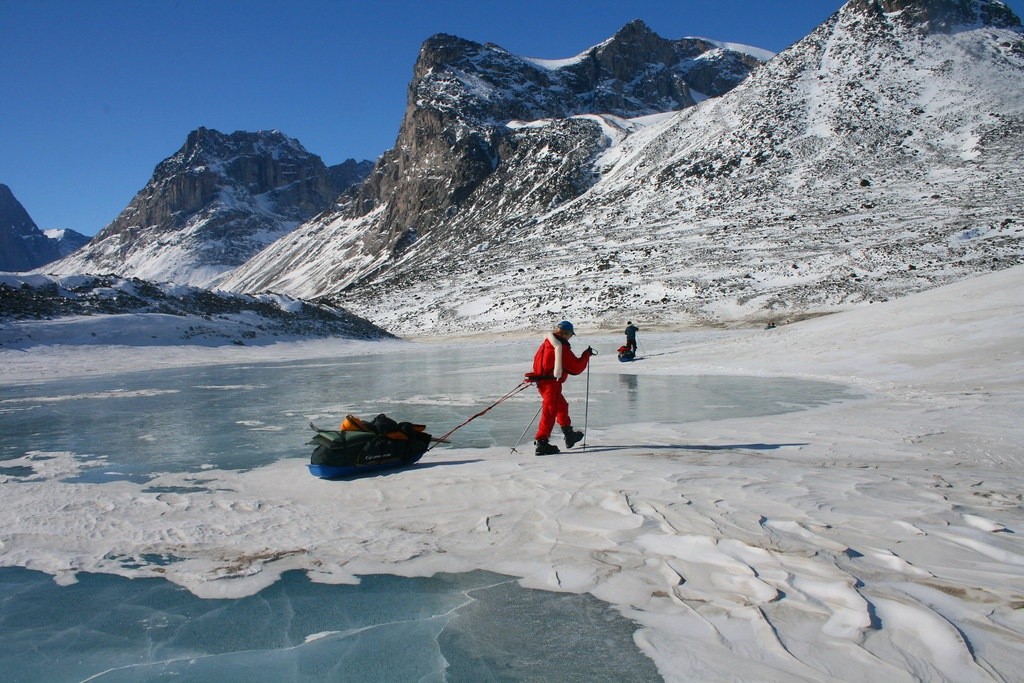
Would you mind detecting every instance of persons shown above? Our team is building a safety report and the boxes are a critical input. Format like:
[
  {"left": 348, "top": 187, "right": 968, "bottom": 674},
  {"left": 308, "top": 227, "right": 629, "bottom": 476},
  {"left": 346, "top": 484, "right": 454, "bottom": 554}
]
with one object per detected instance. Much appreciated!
[
  {"left": 767, "top": 323, "right": 776, "bottom": 329},
  {"left": 533, "top": 322, "right": 593, "bottom": 455},
  {"left": 625, "top": 321, "right": 639, "bottom": 357}
]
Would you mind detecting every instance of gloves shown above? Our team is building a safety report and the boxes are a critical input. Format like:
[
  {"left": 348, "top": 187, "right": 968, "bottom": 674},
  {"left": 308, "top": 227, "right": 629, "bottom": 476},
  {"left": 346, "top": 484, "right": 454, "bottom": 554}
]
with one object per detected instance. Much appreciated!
[{"left": 588, "top": 345, "right": 593, "bottom": 356}]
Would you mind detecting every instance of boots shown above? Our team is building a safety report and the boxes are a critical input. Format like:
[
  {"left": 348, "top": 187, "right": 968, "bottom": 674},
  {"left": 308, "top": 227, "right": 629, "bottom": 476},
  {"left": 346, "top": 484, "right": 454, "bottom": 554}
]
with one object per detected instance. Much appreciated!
[
  {"left": 534, "top": 439, "right": 560, "bottom": 455},
  {"left": 561, "top": 424, "right": 584, "bottom": 448}
]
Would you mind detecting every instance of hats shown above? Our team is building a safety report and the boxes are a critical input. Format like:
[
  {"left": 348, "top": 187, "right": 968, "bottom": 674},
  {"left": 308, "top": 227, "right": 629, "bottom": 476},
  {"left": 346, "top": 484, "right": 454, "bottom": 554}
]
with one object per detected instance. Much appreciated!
[{"left": 557, "top": 321, "right": 576, "bottom": 336}]
